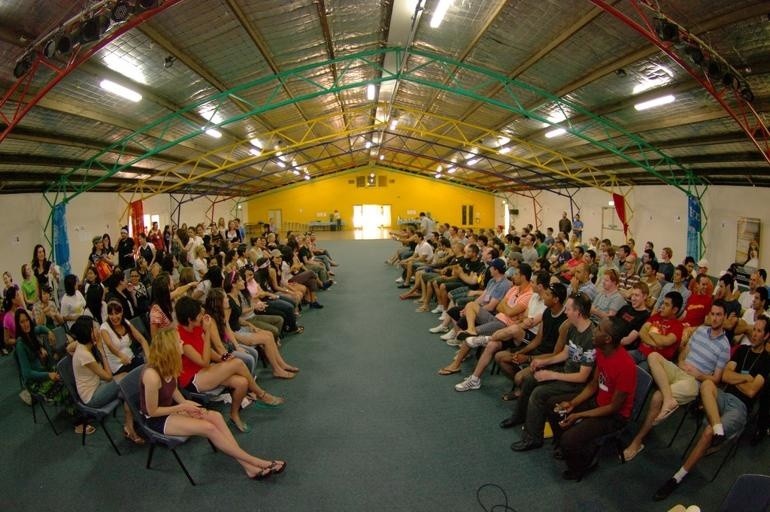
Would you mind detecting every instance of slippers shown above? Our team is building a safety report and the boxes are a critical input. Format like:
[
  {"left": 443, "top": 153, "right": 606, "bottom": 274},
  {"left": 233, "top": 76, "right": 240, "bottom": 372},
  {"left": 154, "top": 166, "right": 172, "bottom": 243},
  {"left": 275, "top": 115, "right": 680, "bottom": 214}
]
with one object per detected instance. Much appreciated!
[
  {"left": 457, "top": 331, "right": 478, "bottom": 341},
  {"left": 619, "top": 444, "right": 645, "bottom": 462},
  {"left": 285, "top": 366, "right": 299, "bottom": 372},
  {"left": 655, "top": 401, "right": 680, "bottom": 422},
  {"left": 439, "top": 367, "right": 462, "bottom": 376},
  {"left": 272, "top": 372, "right": 297, "bottom": 379},
  {"left": 254, "top": 468, "right": 273, "bottom": 481},
  {"left": 265, "top": 460, "right": 287, "bottom": 473}
]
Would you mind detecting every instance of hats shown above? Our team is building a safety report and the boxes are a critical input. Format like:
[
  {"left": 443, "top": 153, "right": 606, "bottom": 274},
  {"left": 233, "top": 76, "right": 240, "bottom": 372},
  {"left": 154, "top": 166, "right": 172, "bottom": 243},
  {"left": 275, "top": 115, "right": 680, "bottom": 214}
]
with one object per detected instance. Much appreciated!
[
  {"left": 487, "top": 259, "right": 507, "bottom": 272},
  {"left": 697, "top": 258, "right": 709, "bottom": 269},
  {"left": 505, "top": 252, "right": 524, "bottom": 263},
  {"left": 271, "top": 249, "right": 284, "bottom": 260}
]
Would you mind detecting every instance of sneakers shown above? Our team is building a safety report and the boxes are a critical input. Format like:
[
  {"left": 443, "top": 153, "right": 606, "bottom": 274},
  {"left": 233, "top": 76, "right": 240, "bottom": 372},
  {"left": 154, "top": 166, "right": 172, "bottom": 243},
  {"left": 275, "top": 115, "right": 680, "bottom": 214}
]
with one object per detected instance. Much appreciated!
[
  {"left": 466, "top": 336, "right": 490, "bottom": 349},
  {"left": 448, "top": 339, "right": 465, "bottom": 347},
  {"left": 19, "top": 390, "right": 33, "bottom": 406},
  {"left": 416, "top": 304, "right": 448, "bottom": 321},
  {"left": 455, "top": 375, "right": 481, "bottom": 392},
  {"left": 395, "top": 278, "right": 404, "bottom": 283},
  {"left": 429, "top": 325, "right": 450, "bottom": 334},
  {"left": 440, "top": 330, "right": 458, "bottom": 341}
]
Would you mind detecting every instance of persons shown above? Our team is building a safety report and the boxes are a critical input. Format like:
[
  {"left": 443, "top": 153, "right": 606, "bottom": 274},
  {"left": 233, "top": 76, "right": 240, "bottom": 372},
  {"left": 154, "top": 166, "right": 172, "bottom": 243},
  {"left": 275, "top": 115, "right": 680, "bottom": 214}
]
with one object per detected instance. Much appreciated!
[
  {"left": 1, "top": 217, "right": 338, "bottom": 479},
  {"left": 385, "top": 208, "right": 769, "bottom": 500}
]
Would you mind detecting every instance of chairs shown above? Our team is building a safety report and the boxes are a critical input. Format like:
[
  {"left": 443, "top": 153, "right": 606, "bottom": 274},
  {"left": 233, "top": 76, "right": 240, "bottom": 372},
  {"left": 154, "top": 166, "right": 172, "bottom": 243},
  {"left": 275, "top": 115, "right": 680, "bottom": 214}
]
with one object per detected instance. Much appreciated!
[
  {"left": 56, "top": 355, "right": 126, "bottom": 457},
  {"left": 715, "top": 471, "right": 770, "bottom": 512},
  {"left": 119, "top": 364, "right": 215, "bottom": 487},
  {"left": 12, "top": 345, "right": 76, "bottom": 436}
]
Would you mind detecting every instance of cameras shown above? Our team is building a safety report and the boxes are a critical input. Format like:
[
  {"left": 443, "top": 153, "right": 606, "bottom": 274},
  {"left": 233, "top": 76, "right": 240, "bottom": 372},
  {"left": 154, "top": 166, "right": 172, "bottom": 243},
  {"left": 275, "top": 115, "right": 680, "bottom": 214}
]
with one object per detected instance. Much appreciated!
[{"left": 559, "top": 410, "right": 567, "bottom": 421}]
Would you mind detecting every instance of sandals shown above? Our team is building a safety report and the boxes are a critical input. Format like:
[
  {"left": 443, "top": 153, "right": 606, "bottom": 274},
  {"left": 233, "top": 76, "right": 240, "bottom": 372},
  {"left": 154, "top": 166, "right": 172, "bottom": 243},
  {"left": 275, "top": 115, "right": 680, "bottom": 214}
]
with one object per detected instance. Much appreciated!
[
  {"left": 257, "top": 392, "right": 286, "bottom": 407},
  {"left": 123, "top": 426, "right": 146, "bottom": 445},
  {"left": 76, "top": 425, "right": 97, "bottom": 435},
  {"left": 230, "top": 418, "right": 252, "bottom": 434}
]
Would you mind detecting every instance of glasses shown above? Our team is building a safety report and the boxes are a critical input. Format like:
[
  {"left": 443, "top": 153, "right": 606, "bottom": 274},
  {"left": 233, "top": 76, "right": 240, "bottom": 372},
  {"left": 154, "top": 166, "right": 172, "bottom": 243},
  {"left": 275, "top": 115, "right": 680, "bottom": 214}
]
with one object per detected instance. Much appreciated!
[{"left": 611, "top": 269, "right": 617, "bottom": 277}]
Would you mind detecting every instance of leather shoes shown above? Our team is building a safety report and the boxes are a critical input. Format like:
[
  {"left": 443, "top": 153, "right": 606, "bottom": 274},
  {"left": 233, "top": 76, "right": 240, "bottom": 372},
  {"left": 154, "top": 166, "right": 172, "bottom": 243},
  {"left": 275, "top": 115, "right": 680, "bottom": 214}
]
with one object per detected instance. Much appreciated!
[
  {"left": 654, "top": 478, "right": 681, "bottom": 501},
  {"left": 555, "top": 452, "right": 566, "bottom": 461},
  {"left": 398, "top": 284, "right": 410, "bottom": 288},
  {"left": 511, "top": 439, "right": 544, "bottom": 452},
  {"left": 562, "top": 470, "right": 581, "bottom": 481},
  {"left": 310, "top": 302, "right": 323, "bottom": 309},
  {"left": 712, "top": 431, "right": 728, "bottom": 448},
  {"left": 500, "top": 418, "right": 516, "bottom": 429},
  {"left": 320, "top": 280, "right": 333, "bottom": 290}
]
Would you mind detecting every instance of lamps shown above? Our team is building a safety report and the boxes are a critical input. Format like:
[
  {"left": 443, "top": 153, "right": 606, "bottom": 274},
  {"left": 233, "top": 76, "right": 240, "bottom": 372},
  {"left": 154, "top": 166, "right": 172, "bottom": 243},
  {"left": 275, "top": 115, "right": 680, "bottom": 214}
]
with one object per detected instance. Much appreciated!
[
  {"left": 164, "top": 55, "right": 177, "bottom": 68},
  {"left": 12, "top": 0, "right": 167, "bottom": 78},
  {"left": 652, "top": 12, "right": 754, "bottom": 102}
]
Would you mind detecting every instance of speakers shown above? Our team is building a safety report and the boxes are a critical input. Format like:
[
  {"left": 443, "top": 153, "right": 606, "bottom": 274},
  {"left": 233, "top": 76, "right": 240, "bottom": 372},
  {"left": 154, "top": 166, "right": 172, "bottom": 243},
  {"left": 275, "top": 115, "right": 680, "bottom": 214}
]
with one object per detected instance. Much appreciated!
[{"left": 509, "top": 209, "right": 519, "bottom": 215}]
